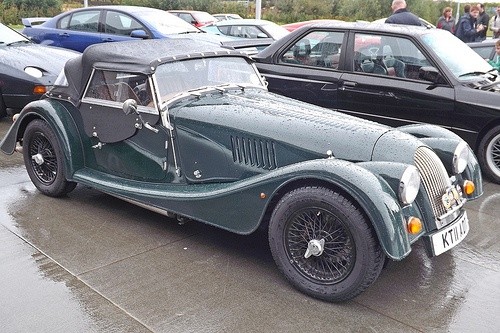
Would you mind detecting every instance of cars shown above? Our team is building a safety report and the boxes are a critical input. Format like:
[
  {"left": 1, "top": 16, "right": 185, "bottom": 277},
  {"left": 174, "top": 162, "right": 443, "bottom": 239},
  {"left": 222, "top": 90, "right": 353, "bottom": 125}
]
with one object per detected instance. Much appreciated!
[
  {"left": 22, "top": 5, "right": 259, "bottom": 54},
  {"left": 200, "top": 18, "right": 318, "bottom": 51},
  {"left": 252, "top": 21, "right": 500, "bottom": 186},
  {"left": 213, "top": 14, "right": 244, "bottom": 22},
  {"left": 0, "top": 22, "right": 83, "bottom": 119},
  {"left": 164, "top": 9, "right": 218, "bottom": 27},
  {"left": 0, "top": 38, "right": 485, "bottom": 304},
  {"left": 281, "top": 19, "right": 500, "bottom": 64}
]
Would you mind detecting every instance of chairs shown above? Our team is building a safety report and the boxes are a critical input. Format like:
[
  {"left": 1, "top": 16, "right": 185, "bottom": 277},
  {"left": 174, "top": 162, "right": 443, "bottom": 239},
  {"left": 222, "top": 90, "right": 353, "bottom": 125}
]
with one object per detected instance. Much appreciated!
[
  {"left": 95, "top": 82, "right": 142, "bottom": 106},
  {"left": 359, "top": 45, "right": 406, "bottom": 79}
]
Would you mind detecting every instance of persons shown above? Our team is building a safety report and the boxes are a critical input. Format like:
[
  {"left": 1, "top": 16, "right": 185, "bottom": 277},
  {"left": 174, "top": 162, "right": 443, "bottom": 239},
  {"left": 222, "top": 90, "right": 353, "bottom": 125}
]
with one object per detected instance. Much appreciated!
[
  {"left": 380, "top": 0, "right": 421, "bottom": 61},
  {"left": 454, "top": 3, "right": 489, "bottom": 44},
  {"left": 489, "top": 6, "right": 500, "bottom": 39},
  {"left": 485, "top": 40, "right": 500, "bottom": 72},
  {"left": 436, "top": 7, "right": 455, "bottom": 35}
]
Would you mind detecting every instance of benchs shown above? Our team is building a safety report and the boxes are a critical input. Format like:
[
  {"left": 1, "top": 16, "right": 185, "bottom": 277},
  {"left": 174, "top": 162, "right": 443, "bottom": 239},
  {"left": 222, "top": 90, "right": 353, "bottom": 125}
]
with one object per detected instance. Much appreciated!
[{"left": 284, "top": 38, "right": 340, "bottom": 70}]
